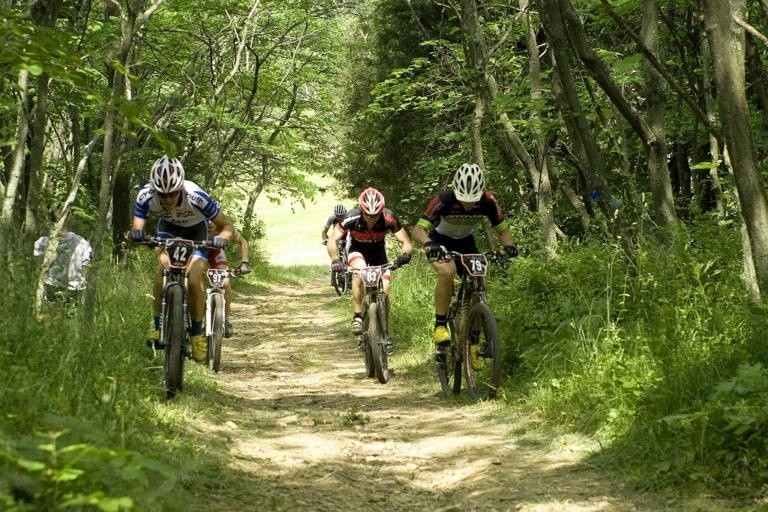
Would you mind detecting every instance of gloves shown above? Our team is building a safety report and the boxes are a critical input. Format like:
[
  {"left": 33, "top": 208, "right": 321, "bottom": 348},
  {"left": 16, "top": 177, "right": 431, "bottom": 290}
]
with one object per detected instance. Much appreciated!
[
  {"left": 235, "top": 262, "right": 252, "bottom": 276},
  {"left": 503, "top": 245, "right": 518, "bottom": 258},
  {"left": 397, "top": 253, "right": 411, "bottom": 265},
  {"left": 128, "top": 228, "right": 144, "bottom": 242},
  {"left": 212, "top": 236, "right": 224, "bottom": 248},
  {"left": 424, "top": 241, "right": 447, "bottom": 263},
  {"left": 332, "top": 259, "right": 344, "bottom": 272}
]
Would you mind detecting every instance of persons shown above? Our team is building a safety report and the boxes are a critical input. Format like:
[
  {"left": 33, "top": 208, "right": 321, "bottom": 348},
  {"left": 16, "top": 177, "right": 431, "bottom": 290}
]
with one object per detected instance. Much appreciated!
[
  {"left": 408, "top": 160, "right": 518, "bottom": 372},
  {"left": 320, "top": 202, "right": 351, "bottom": 281},
  {"left": 31, "top": 201, "right": 93, "bottom": 317},
  {"left": 327, "top": 186, "right": 413, "bottom": 356},
  {"left": 195, "top": 209, "right": 250, "bottom": 340},
  {"left": 125, "top": 155, "right": 235, "bottom": 364}
]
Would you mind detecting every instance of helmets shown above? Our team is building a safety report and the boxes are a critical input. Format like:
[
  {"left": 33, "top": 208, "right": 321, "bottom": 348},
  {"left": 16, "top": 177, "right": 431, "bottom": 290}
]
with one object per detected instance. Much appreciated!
[
  {"left": 451, "top": 163, "right": 486, "bottom": 203},
  {"left": 358, "top": 187, "right": 385, "bottom": 217},
  {"left": 150, "top": 154, "right": 185, "bottom": 194},
  {"left": 334, "top": 204, "right": 347, "bottom": 216}
]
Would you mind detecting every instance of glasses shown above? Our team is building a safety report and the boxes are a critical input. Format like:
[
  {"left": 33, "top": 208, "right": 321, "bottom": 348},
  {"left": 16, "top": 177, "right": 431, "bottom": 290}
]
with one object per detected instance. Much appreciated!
[{"left": 157, "top": 190, "right": 180, "bottom": 199}]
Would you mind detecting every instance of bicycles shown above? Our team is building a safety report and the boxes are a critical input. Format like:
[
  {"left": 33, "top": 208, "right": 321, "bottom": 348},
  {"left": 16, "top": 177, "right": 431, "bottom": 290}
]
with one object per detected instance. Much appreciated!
[
  {"left": 321, "top": 239, "right": 353, "bottom": 297},
  {"left": 333, "top": 257, "right": 402, "bottom": 383},
  {"left": 117, "top": 230, "right": 227, "bottom": 400},
  {"left": 417, "top": 243, "right": 515, "bottom": 397},
  {"left": 199, "top": 266, "right": 250, "bottom": 376}
]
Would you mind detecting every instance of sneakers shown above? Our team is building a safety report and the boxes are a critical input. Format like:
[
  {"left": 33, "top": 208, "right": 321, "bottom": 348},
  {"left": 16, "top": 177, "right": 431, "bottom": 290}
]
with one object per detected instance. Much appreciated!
[
  {"left": 191, "top": 334, "right": 208, "bottom": 362},
  {"left": 225, "top": 320, "right": 233, "bottom": 338},
  {"left": 434, "top": 326, "right": 451, "bottom": 346},
  {"left": 471, "top": 345, "right": 484, "bottom": 371},
  {"left": 147, "top": 330, "right": 166, "bottom": 350},
  {"left": 350, "top": 312, "right": 363, "bottom": 332}
]
